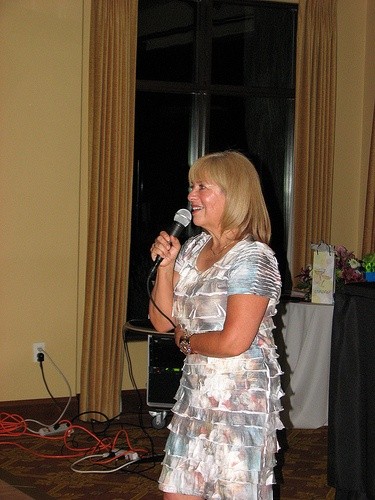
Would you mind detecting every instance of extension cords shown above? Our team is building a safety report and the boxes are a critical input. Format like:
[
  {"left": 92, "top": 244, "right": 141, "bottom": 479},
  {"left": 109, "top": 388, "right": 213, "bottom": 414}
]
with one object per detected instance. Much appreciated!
[
  {"left": 40, "top": 423, "right": 71, "bottom": 437},
  {"left": 111, "top": 448, "right": 138, "bottom": 461}
]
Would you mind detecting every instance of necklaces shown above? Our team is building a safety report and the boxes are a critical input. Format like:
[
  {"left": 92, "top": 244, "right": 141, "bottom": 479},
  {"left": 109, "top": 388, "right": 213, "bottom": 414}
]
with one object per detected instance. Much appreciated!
[{"left": 210, "top": 241, "right": 235, "bottom": 255}]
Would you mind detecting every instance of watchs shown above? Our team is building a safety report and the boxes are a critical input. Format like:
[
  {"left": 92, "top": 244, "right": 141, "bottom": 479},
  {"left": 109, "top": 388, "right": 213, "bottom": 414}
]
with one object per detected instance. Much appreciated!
[{"left": 179, "top": 331, "right": 194, "bottom": 355}]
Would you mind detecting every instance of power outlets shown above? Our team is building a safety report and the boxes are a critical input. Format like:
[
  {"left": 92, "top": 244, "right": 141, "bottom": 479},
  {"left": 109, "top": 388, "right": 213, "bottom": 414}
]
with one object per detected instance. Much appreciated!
[{"left": 33, "top": 343, "right": 47, "bottom": 363}]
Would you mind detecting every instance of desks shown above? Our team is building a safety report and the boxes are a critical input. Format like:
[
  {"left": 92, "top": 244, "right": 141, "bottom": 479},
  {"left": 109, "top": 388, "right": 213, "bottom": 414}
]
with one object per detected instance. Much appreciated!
[{"left": 273, "top": 299, "right": 329, "bottom": 429}]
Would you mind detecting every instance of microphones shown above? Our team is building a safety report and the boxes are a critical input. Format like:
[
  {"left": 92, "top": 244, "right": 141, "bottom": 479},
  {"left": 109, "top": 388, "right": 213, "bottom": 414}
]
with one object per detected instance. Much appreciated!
[{"left": 151, "top": 209, "right": 192, "bottom": 273}]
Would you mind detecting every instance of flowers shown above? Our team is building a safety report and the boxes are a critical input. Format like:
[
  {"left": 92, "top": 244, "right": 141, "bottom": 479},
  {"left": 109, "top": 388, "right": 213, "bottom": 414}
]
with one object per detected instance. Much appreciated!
[{"left": 294, "top": 238, "right": 375, "bottom": 302}]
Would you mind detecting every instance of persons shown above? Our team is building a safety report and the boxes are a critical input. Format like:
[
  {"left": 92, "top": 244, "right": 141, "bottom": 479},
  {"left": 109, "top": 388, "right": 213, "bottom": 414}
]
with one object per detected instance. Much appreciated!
[{"left": 151, "top": 153, "right": 286, "bottom": 500}]
